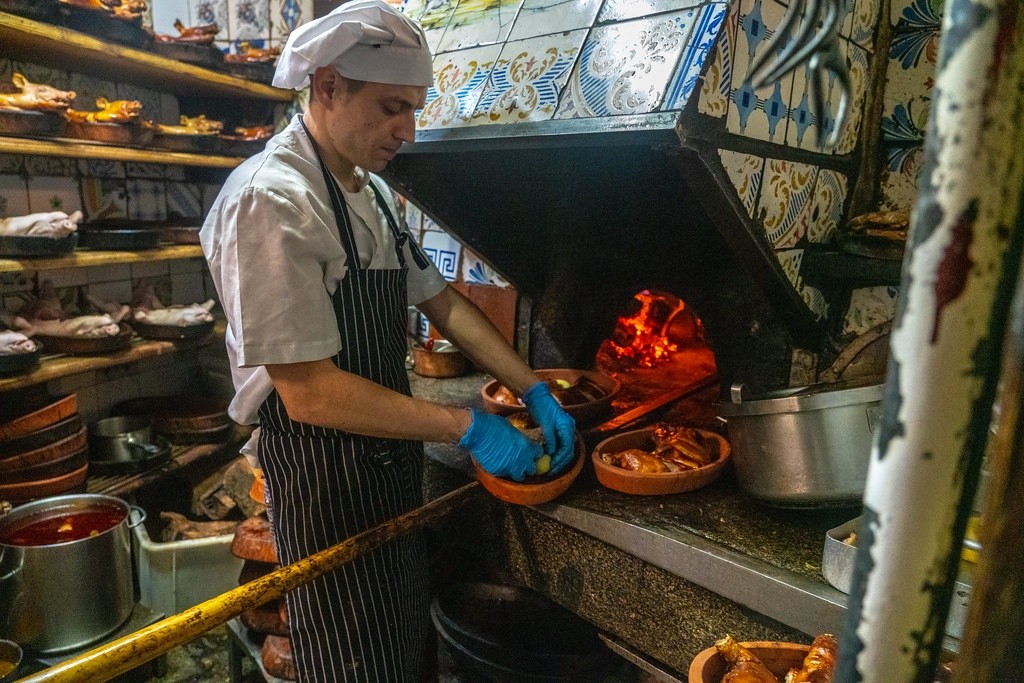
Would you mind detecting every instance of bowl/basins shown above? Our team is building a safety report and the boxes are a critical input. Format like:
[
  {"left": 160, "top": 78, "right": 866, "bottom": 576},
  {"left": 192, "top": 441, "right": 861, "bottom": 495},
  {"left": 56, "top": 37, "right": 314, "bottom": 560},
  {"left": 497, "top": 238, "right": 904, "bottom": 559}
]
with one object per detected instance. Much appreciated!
[
  {"left": 410, "top": 339, "right": 473, "bottom": 378},
  {"left": 591, "top": 427, "right": 731, "bottom": 496},
  {"left": 469, "top": 408, "right": 586, "bottom": 505},
  {"left": 481, "top": 369, "right": 620, "bottom": 425}
]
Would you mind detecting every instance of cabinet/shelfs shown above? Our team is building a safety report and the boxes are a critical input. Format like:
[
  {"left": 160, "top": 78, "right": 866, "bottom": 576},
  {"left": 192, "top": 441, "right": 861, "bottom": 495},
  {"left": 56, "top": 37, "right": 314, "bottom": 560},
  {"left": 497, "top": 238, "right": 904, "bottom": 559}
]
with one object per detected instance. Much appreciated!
[{"left": 0, "top": 10, "right": 294, "bottom": 504}]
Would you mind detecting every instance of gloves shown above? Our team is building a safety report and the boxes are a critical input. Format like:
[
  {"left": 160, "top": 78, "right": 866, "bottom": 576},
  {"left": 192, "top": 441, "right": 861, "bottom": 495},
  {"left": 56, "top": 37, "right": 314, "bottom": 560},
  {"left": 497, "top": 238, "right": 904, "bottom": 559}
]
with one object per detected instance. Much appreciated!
[
  {"left": 456, "top": 407, "right": 543, "bottom": 482},
  {"left": 521, "top": 382, "right": 578, "bottom": 475}
]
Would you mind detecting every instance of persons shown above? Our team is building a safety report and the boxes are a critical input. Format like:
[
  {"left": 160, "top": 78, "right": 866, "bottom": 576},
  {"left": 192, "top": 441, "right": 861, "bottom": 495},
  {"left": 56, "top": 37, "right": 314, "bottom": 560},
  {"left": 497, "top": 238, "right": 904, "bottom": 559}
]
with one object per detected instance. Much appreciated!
[{"left": 197, "top": 0, "right": 577, "bottom": 682}]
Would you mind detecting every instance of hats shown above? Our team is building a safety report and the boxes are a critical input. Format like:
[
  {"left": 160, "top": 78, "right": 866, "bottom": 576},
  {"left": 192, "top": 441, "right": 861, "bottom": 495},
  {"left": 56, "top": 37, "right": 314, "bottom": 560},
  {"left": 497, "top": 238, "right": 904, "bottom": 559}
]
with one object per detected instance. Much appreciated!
[{"left": 271, "top": 0, "right": 434, "bottom": 91}]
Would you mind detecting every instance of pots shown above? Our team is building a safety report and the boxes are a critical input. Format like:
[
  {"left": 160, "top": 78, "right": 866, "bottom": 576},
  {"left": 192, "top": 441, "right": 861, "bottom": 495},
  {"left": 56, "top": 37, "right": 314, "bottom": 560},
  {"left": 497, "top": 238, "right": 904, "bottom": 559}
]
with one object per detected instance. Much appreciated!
[
  {"left": 85, "top": 414, "right": 161, "bottom": 464},
  {"left": 430, "top": 578, "right": 612, "bottom": 683},
  {"left": 712, "top": 381, "right": 887, "bottom": 511},
  {"left": 0, "top": 493, "right": 147, "bottom": 655}
]
